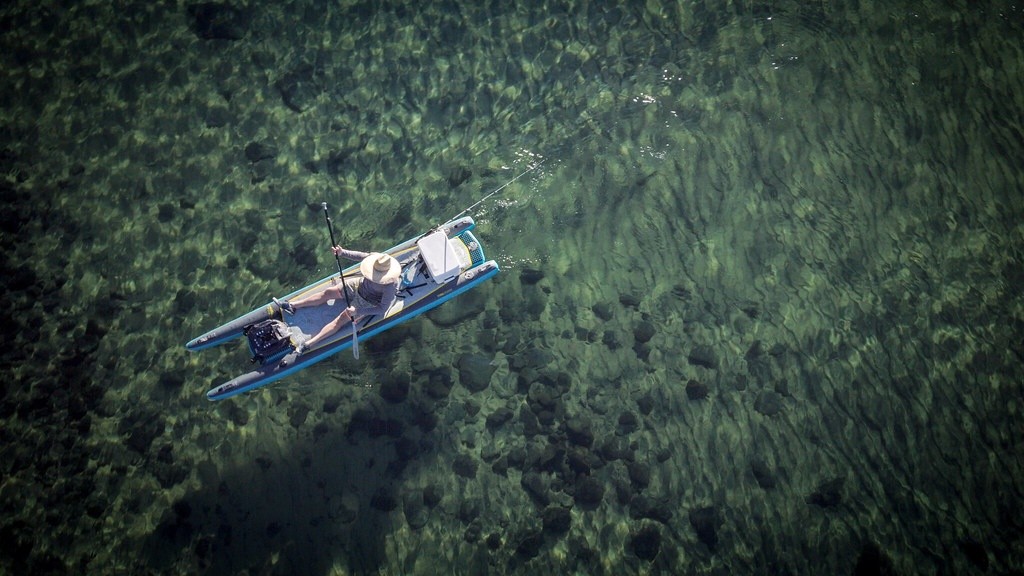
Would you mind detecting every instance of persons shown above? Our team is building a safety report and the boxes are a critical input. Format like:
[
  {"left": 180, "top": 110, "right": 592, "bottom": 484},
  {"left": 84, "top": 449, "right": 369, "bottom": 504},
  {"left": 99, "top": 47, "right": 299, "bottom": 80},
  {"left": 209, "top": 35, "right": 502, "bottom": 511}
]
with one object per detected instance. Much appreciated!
[{"left": 272, "top": 245, "right": 401, "bottom": 367}]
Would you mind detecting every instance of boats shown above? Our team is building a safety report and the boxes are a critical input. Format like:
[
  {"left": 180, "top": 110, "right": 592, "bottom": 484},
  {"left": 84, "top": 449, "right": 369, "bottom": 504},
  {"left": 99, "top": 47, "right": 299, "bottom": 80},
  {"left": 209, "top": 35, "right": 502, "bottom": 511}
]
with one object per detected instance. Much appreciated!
[{"left": 182, "top": 213, "right": 498, "bottom": 405}]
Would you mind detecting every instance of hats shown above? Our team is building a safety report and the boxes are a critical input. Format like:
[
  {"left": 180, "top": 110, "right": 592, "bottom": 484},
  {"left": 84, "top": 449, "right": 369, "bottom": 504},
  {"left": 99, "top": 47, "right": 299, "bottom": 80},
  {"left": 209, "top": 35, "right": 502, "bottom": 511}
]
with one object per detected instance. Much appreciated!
[{"left": 360, "top": 253, "right": 400, "bottom": 285}]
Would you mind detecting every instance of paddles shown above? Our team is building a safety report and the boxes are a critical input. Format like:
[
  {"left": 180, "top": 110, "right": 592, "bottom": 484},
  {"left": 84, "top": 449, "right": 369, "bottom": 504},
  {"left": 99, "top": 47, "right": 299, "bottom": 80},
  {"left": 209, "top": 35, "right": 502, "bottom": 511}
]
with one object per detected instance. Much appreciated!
[{"left": 322, "top": 202, "right": 359, "bottom": 360}]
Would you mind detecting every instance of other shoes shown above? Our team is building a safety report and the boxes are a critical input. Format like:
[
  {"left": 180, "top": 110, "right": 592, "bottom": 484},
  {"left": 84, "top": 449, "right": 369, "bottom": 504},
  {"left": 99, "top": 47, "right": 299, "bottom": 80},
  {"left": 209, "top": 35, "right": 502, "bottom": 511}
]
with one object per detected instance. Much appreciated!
[
  {"left": 296, "top": 345, "right": 307, "bottom": 357},
  {"left": 273, "top": 297, "right": 295, "bottom": 315}
]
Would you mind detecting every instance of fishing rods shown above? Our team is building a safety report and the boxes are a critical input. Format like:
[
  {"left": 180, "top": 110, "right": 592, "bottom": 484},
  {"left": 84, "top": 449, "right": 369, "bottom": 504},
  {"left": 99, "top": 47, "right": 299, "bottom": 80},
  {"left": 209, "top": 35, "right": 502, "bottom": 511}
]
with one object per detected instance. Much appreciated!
[{"left": 415, "top": 157, "right": 549, "bottom": 244}]
